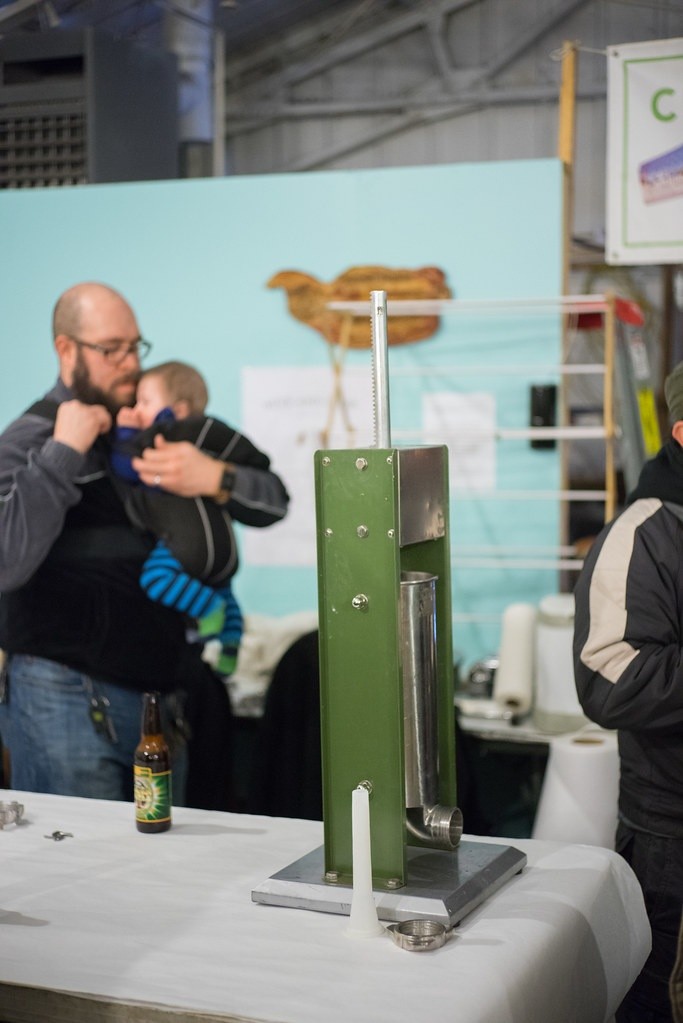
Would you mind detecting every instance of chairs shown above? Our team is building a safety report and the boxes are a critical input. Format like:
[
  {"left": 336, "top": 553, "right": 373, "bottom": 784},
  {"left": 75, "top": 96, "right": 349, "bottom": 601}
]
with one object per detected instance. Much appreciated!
[{"left": 225, "top": 632, "right": 320, "bottom": 819}]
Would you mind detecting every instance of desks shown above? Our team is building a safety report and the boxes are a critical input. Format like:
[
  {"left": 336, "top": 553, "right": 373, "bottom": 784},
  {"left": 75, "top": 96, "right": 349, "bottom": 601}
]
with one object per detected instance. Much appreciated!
[{"left": 0, "top": 786, "right": 651, "bottom": 1023}]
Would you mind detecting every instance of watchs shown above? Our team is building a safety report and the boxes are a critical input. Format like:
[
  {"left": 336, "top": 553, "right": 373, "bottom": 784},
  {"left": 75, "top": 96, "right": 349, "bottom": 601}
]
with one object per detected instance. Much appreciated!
[{"left": 212, "top": 460, "right": 235, "bottom": 503}]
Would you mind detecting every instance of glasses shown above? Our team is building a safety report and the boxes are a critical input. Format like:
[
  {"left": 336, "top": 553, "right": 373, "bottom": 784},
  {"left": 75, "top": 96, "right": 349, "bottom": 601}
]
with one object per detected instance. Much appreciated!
[{"left": 71, "top": 339, "right": 151, "bottom": 365}]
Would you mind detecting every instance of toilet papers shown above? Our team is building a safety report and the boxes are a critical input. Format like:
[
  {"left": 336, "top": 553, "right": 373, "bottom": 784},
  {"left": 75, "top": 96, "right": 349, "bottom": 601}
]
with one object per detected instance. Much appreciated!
[{"left": 493, "top": 602, "right": 535, "bottom": 715}]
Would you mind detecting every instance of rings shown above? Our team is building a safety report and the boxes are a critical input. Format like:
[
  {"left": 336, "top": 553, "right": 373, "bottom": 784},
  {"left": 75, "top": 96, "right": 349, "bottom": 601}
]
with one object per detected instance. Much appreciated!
[{"left": 154, "top": 473, "right": 160, "bottom": 486}]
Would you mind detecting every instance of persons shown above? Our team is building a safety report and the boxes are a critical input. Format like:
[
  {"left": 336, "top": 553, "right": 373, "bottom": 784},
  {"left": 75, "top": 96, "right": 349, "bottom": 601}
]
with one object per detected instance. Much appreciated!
[
  {"left": 574, "top": 361, "right": 683, "bottom": 1023},
  {"left": 111, "top": 361, "right": 244, "bottom": 677},
  {"left": 0, "top": 282, "right": 287, "bottom": 806}
]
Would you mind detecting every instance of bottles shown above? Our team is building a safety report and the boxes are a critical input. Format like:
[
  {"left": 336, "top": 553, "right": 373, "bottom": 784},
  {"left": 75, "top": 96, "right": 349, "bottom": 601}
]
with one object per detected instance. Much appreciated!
[{"left": 134, "top": 691, "right": 171, "bottom": 833}]
